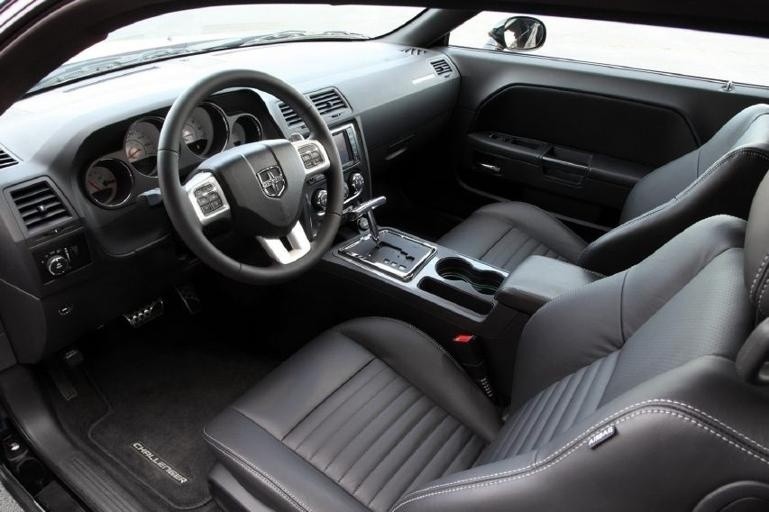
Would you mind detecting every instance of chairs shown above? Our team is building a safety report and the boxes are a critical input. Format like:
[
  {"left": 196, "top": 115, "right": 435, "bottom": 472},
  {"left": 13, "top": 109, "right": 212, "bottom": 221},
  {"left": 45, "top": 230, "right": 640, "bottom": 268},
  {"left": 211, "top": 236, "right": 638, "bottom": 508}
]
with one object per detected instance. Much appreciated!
[
  {"left": 437, "top": 102, "right": 768, "bottom": 269},
  {"left": 202, "top": 175, "right": 768, "bottom": 512}
]
